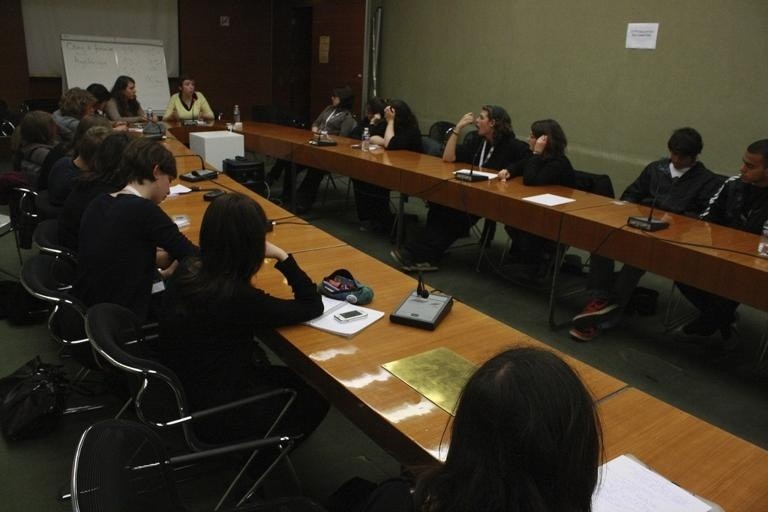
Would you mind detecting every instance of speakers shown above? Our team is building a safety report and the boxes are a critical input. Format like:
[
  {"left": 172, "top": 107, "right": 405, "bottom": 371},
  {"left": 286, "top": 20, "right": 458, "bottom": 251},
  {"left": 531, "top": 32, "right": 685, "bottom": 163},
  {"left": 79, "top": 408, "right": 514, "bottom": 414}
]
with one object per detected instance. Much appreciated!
[{"left": 222, "top": 159, "right": 265, "bottom": 196}]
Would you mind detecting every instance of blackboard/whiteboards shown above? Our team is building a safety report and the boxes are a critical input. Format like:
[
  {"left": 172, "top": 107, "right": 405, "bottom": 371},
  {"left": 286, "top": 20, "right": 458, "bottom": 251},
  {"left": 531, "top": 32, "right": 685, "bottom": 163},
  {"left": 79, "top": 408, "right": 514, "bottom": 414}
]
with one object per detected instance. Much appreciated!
[{"left": 60, "top": 33, "right": 171, "bottom": 116}]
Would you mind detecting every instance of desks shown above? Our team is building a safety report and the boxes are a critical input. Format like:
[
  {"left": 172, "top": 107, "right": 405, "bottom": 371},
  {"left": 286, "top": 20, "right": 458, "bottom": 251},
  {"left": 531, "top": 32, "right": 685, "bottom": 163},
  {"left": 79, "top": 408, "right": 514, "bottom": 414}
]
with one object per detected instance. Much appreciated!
[
  {"left": 592, "top": 385, "right": 768, "bottom": 512},
  {"left": 157, "top": 118, "right": 292, "bottom": 243},
  {"left": 237, "top": 119, "right": 418, "bottom": 232},
  {"left": 401, "top": 153, "right": 618, "bottom": 331},
  {"left": 549, "top": 201, "right": 766, "bottom": 335},
  {"left": 249, "top": 235, "right": 623, "bottom": 468}
]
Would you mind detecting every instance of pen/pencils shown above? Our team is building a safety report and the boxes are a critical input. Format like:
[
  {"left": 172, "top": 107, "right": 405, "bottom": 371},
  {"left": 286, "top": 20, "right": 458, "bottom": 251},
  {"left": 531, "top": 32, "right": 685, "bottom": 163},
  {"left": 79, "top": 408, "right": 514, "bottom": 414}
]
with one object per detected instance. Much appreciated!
[{"left": 322, "top": 275, "right": 354, "bottom": 295}]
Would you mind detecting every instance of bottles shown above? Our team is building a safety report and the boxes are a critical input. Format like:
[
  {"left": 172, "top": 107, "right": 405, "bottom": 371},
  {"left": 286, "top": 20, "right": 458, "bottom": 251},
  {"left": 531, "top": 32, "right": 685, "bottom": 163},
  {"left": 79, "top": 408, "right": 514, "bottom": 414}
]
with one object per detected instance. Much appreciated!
[
  {"left": 146, "top": 107, "right": 153, "bottom": 123},
  {"left": 756, "top": 221, "right": 768, "bottom": 257},
  {"left": 361, "top": 127, "right": 370, "bottom": 153},
  {"left": 234, "top": 106, "right": 240, "bottom": 123}
]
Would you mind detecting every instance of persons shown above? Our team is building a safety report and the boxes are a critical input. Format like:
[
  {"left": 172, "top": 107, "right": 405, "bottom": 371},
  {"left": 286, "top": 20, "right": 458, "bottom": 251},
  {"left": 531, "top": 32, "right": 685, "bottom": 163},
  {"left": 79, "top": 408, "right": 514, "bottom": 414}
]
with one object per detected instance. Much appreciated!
[
  {"left": 358, "top": 345, "right": 603, "bottom": 512},
  {"left": 156, "top": 192, "right": 331, "bottom": 504}
]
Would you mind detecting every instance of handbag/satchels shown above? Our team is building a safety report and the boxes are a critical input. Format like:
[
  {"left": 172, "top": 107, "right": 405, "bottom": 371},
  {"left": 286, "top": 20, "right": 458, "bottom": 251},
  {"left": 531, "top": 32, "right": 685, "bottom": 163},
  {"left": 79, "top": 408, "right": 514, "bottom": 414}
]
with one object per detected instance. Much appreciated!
[
  {"left": 0, "top": 280, "right": 43, "bottom": 326},
  {"left": 0, "top": 355, "right": 108, "bottom": 444}
]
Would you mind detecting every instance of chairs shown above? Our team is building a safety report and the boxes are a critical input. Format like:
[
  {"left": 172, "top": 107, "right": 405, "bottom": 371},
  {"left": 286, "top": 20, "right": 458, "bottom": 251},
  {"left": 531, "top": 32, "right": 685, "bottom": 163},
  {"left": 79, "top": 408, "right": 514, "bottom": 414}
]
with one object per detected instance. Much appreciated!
[
  {"left": 429, "top": 120, "right": 456, "bottom": 141},
  {"left": 61, "top": 301, "right": 304, "bottom": 511},
  {"left": 11, "top": 188, "right": 160, "bottom": 368}
]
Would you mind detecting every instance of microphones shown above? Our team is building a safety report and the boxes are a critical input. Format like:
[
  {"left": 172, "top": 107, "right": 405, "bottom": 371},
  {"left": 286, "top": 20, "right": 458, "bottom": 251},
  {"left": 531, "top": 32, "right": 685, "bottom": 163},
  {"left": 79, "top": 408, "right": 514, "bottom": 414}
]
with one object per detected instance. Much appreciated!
[
  {"left": 390, "top": 273, "right": 452, "bottom": 331},
  {"left": 183, "top": 101, "right": 198, "bottom": 126},
  {"left": 127, "top": 107, "right": 165, "bottom": 141},
  {"left": 627, "top": 176, "right": 670, "bottom": 232},
  {"left": 190, "top": 170, "right": 272, "bottom": 233},
  {"left": 308, "top": 112, "right": 349, "bottom": 146},
  {"left": 455, "top": 136, "right": 489, "bottom": 181}
]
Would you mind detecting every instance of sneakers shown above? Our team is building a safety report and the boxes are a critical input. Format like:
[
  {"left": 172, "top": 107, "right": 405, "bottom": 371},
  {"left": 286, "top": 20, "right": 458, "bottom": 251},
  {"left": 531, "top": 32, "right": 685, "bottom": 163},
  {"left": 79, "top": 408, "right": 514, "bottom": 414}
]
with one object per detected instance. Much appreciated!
[
  {"left": 401, "top": 256, "right": 441, "bottom": 273},
  {"left": 683, "top": 311, "right": 741, "bottom": 336},
  {"left": 568, "top": 295, "right": 620, "bottom": 341}
]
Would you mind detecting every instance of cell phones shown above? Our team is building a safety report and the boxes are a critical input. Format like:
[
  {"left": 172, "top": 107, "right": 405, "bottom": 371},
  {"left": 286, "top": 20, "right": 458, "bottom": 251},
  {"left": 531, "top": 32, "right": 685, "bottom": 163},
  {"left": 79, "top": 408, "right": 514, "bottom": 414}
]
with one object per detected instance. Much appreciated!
[
  {"left": 204, "top": 190, "right": 223, "bottom": 201},
  {"left": 334, "top": 309, "right": 368, "bottom": 323}
]
